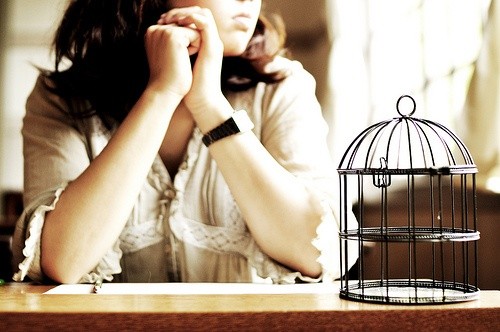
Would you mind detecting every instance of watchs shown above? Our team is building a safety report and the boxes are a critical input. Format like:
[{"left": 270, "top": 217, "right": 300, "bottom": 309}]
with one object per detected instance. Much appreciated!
[{"left": 202, "top": 109, "right": 254, "bottom": 148}]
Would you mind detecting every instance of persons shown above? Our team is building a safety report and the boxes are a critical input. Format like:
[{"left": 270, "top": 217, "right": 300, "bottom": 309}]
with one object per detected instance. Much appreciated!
[{"left": 10, "top": 0, "right": 364, "bottom": 285}]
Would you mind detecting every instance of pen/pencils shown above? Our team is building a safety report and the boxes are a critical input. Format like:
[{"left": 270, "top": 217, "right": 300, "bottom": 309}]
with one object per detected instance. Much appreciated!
[{"left": 93, "top": 279, "right": 102, "bottom": 293}]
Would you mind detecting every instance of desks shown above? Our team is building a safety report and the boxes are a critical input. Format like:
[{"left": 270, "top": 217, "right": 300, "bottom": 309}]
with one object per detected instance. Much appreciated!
[{"left": 0, "top": 282, "right": 499, "bottom": 332}]
